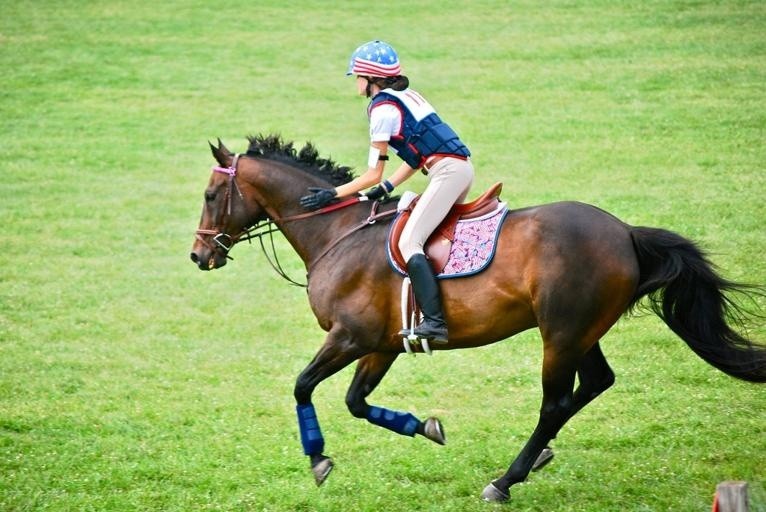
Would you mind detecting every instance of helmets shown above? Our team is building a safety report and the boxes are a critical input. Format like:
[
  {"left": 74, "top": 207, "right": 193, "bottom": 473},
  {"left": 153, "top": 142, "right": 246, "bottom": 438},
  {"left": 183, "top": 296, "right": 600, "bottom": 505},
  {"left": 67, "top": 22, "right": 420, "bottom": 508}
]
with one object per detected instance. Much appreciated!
[{"left": 345, "top": 41, "right": 401, "bottom": 78}]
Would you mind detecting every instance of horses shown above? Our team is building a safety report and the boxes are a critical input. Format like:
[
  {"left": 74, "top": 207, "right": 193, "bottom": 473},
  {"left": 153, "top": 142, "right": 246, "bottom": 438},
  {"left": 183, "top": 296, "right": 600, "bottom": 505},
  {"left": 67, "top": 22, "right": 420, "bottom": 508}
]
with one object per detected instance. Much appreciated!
[{"left": 189, "top": 132, "right": 766, "bottom": 503}]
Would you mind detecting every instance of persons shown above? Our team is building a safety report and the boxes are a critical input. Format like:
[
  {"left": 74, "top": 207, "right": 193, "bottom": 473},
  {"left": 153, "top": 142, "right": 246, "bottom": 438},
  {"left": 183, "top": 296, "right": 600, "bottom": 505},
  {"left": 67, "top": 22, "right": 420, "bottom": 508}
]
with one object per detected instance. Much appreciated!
[{"left": 297, "top": 37, "right": 478, "bottom": 350}]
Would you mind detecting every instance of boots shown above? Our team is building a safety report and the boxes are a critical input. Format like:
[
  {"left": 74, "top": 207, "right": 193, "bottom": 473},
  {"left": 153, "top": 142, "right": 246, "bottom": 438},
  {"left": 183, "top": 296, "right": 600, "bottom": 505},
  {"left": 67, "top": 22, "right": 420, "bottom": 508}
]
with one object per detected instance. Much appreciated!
[{"left": 398, "top": 253, "right": 449, "bottom": 347}]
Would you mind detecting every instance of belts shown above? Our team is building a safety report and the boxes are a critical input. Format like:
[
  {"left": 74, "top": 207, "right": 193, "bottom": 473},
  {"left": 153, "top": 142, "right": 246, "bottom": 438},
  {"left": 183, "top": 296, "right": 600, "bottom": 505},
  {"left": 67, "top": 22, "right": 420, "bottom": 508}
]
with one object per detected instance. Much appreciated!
[{"left": 421, "top": 154, "right": 446, "bottom": 175}]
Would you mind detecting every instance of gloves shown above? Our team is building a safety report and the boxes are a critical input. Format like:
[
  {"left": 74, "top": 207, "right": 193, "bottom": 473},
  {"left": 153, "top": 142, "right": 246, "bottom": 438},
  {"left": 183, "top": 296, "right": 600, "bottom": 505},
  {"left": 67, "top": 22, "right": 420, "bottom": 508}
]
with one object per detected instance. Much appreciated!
[
  {"left": 299, "top": 187, "right": 341, "bottom": 211},
  {"left": 365, "top": 179, "right": 395, "bottom": 200}
]
